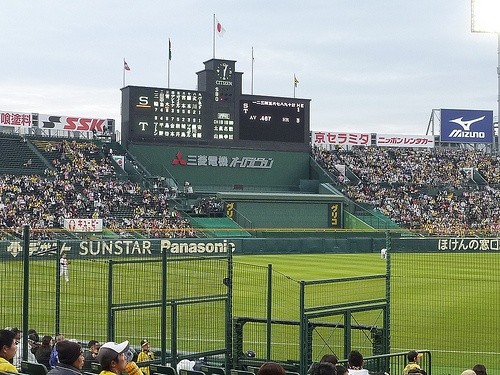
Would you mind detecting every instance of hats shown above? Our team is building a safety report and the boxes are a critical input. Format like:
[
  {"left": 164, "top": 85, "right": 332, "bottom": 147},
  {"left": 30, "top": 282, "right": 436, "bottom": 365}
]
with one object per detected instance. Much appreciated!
[
  {"left": 56, "top": 340, "right": 83, "bottom": 364},
  {"left": 98, "top": 340, "right": 129, "bottom": 368},
  {"left": 407, "top": 350, "right": 423, "bottom": 360},
  {"left": 473, "top": 364, "right": 487, "bottom": 375},
  {"left": 10, "top": 327, "right": 21, "bottom": 333},
  {"left": 141, "top": 339, "right": 150, "bottom": 347},
  {"left": 176, "top": 359, "right": 195, "bottom": 374}
]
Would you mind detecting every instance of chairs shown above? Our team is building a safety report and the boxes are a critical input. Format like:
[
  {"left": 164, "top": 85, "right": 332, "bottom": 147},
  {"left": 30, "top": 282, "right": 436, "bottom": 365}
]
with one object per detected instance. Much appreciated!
[
  {"left": 199, "top": 365, "right": 226, "bottom": 375},
  {"left": 231, "top": 369, "right": 255, "bottom": 375},
  {"left": 149, "top": 364, "right": 176, "bottom": 375},
  {"left": 20, "top": 361, "right": 49, "bottom": 375},
  {"left": 247, "top": 366, "right": 260, "bottom": 375},
  {"left": 91, "top": 363, "right": 103, "bottom": 374},
  {"left": 179, "top": 369, "right": 206, "bottom": 375}
]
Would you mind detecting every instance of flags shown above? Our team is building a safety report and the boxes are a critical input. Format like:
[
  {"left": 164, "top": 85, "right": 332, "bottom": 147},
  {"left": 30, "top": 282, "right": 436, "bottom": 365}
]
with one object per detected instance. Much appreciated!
[
  {"left": 294, "top": 76, "right": 299, "bottom": 87},
  {"left": 169, "top": 41, "right": 172, "bottom": 60},
  {"left": 216, "top": 17, "right": 225, "bottom": 36},
  {"left": 125, "top": 61, "right": 130, "bottom": 70}
]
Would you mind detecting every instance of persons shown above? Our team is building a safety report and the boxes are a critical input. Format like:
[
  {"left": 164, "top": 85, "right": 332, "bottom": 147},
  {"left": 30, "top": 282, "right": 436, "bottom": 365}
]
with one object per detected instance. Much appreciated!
[
  {"left": 402, "top": 350, "right": 427, "bottom": 375},
  {"left": 0, "top": 140, "right": 222, "bottom": 239},
  {"left": 461, "top": 364, "right": 487, "bottom": 375},
  {"left": 311, "top": 142, "right": 500, "bottom": 237},
  {"left": 0, "top": 326, "right": 154, "bottom": 375},
  {"left": 309, "top": 350, "right": 369, "bottom": 375},
  {"left": 257, "top": 362, "right": 285, "bottom": 375},
  {"left": 177, "top": 359, "right": 195, "bottom": 375},
  {"left": 60, "top": 253, "right": 70, "bottom": 283}
]
